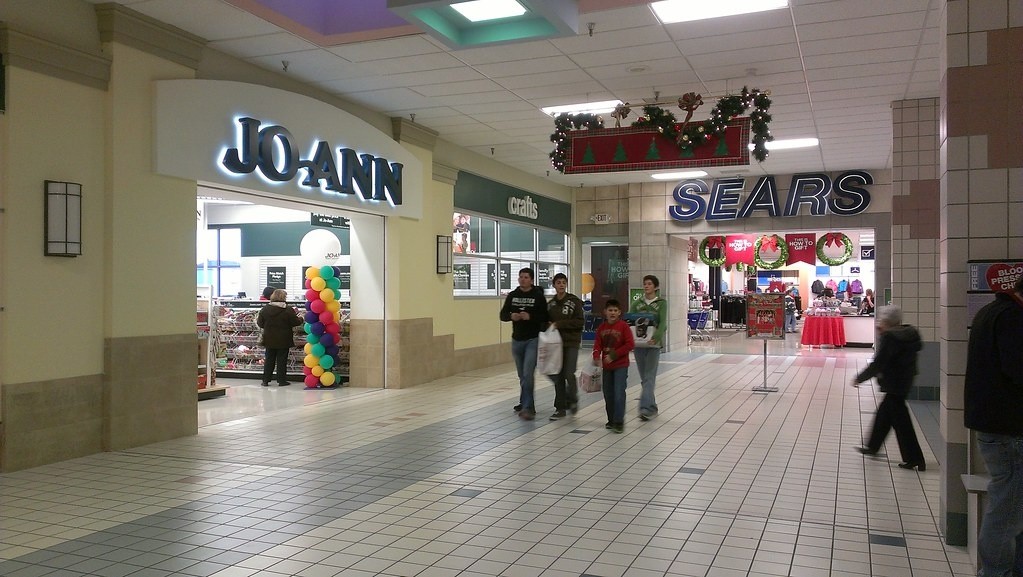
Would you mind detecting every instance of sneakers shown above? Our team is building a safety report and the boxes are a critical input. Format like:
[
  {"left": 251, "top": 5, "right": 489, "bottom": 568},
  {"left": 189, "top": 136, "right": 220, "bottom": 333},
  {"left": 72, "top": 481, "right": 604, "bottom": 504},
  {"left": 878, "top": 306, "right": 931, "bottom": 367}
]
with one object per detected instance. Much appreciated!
[
  {"left": 514, "top": 403, "right": 523, "bottom": 411},
  {"left": 568, "top": 402, "right": 577, "bottom": 414},
  {"left": 520, "top": 410, "right": 537, "bottom": 419},
  {"left": 550, "top": 409, "right": 566, "bottom": 419}
]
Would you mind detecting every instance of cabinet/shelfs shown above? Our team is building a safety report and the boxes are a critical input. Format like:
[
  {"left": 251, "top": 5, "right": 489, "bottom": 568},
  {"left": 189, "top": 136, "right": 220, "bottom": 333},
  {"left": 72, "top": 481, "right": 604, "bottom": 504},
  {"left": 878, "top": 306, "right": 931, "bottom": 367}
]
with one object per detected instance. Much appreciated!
[
  {"left": 196, "top": 284, "right": 226, "bottom": 400},
  {"left": 217, "top": 301, "right": 349, "bottom": 383}
]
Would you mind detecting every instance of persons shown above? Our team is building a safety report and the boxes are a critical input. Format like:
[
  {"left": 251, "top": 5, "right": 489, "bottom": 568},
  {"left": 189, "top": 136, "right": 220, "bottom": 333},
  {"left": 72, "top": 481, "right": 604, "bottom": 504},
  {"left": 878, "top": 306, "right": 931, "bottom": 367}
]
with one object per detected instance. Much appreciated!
[
  {"left": 259, "top": 287, "right": 276, "bottom": 300},
  {"left": 593, "top": 300, "right": 634, "bottom": 433},
  {"left": 785, "top": 290, "right": 799, "bottom": 333},
  {"left": 499, "top": 268, "right": 548, "bottom": 420},
  {"left": 964, "top": 275, "right": 1023, "bottom": 577},
  {"left": 453, "top": 215, "right": 470, "bottom": 251},
  {"left": 853, "top": 305, "right": 926, "bottom": 471},
  {"left": 757, "top": 310, "right": 773, "bottom": 324},
  {"left": 257, "top": 289, "right": 303, "bottom": 386},
  {"left": 547, "top": 273, "right": 585, "bottom": 419},
  {"left": 630, "top": 275, "right": 667, "bottom": 420},
  {"left": 862, "top": 289, "right": 874, "bottom": 313}
]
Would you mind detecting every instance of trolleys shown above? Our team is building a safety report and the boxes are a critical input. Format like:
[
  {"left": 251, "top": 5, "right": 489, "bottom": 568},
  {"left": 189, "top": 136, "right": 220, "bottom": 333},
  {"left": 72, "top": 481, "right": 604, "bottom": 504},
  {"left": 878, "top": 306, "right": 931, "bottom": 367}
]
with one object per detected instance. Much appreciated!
[{"left": 688, "top": 308, "right": 712, "bottom": 345}]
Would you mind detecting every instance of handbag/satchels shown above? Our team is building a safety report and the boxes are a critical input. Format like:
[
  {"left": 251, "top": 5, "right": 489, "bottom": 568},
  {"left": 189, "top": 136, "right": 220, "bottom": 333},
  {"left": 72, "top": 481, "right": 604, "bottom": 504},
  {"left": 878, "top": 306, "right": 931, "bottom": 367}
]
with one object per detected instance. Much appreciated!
[
  {"left": 536, "top": 322, "right": 563, "bottom": 375},
  {"left": 257, "top": 328, "right": 263, "bottom": 344},
  {"left": 580, "top": 361, "right": 602, "bottom": 393}
]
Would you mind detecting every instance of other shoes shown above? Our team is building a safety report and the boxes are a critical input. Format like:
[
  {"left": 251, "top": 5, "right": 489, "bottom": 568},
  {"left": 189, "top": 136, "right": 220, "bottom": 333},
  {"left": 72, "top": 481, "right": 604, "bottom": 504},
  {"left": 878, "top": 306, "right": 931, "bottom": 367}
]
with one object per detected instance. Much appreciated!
[
  {"left": 613, "top": 427, "right": 623, "bottom": 433},
  {"left": 641, "top": 414, "right": 654, "bottom": 421},
  {"left": 261, "top": 381, "right": 268, "bottom": 386},
  {"left": 279, "top": 381, "right": 291, "bottom": 386},
  {"left": 606, "top": 422, "right": 614, "bottom": 430}
]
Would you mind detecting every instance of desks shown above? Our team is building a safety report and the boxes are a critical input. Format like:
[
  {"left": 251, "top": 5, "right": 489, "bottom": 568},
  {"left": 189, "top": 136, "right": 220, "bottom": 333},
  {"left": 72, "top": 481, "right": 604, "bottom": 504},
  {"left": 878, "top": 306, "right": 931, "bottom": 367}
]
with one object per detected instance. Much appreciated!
[{"left": 800, "top": 316, "right": 846, "bottom": 350}]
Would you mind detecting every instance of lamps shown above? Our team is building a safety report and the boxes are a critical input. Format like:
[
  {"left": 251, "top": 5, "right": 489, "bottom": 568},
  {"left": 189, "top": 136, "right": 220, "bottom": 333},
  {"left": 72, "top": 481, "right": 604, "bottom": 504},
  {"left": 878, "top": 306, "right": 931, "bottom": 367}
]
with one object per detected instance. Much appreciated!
[
  {"left": 435, "top": 234, "right": 453, "bottom": 275},
  {"left": 45, "top": 180, "right": 85, "bottom": 260}
]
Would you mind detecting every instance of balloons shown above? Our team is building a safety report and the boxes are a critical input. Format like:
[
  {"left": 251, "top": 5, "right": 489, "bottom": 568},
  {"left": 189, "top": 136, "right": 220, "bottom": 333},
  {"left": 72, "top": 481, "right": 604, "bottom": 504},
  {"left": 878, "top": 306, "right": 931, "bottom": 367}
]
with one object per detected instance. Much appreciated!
[{"left": 300, "top": 229, "right": 342, "bottom": 387}]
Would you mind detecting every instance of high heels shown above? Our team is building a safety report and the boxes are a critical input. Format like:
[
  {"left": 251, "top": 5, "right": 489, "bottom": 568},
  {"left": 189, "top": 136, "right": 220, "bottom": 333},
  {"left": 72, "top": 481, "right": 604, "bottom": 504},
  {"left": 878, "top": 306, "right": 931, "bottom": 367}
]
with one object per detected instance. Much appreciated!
[
  {"left": 898, "top": 457, "right": 926, "bottom": 471},
  {"left": 857, "top": 445, "right": 877, "bottom": 456}
]
